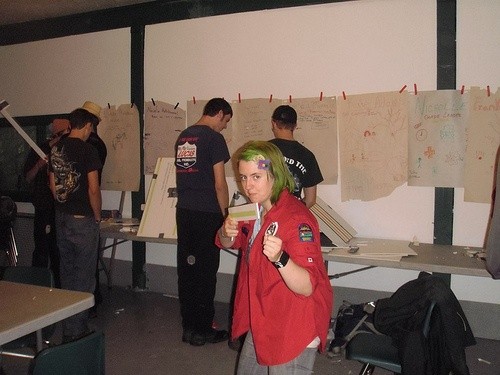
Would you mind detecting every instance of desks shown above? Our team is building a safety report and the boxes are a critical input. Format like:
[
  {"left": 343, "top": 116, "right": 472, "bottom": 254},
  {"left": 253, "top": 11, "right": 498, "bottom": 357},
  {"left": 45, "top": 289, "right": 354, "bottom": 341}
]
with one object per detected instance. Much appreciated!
[
  {"left": 94, "top": 217, "right": 492, "bottom": 288},
  {"left": 0, "top": 280, "right": 96, "bottom": 359}
]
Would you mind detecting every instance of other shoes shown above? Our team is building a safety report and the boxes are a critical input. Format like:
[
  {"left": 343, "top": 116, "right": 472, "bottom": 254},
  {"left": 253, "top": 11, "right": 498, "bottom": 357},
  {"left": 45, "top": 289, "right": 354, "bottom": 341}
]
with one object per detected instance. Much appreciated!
[
  {"left": 181, "top": 328, "right": 229, "bottom": 345},
  {"left": 62, "top": 329, "right": 96, "bottom": 344},
  {"left": 88, "top": 310, "right": 97, "bottom": 318}
]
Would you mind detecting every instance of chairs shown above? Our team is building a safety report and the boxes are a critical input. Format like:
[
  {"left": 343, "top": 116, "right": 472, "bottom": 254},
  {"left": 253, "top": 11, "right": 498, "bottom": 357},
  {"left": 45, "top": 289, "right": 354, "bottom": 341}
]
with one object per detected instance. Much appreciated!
[
  {"left": 0, "top": 266, "right": 56, "bottom": 375},
  {"left": 326, "top": 272, "right": 476, "bottom": 375},
  {"left": 30, "top": 329, "right": 106, "bottom": 375}
]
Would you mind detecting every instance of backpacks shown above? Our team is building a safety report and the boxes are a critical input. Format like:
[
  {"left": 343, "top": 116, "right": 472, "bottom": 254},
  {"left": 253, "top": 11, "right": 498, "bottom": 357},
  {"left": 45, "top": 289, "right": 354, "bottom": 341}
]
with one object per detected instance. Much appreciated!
[{"left": 327, "top": 298, "right": 391, "bottom": 352}]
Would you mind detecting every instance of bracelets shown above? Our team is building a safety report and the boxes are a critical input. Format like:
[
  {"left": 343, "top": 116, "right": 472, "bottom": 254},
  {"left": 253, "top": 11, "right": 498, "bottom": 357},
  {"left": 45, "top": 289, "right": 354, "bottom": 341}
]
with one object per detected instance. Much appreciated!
[
  {"left": 274, "top": 250, "right": 290, "bottom": 269},
  {"left": 96, "top": 218, "right": 100, "bottom": 223},
  {"left": 219, "top": 227, "right": 226, "bottom": 239}
]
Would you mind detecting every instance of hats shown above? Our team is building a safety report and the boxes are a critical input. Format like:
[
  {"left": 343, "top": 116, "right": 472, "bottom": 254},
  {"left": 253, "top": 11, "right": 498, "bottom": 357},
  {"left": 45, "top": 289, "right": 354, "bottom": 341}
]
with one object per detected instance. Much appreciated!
[
  {"left": 81, "top": 101, "right": 103, "bottom": 123},
  {"left": 49, "top": 119, "right": 71, "bottom": 135}
]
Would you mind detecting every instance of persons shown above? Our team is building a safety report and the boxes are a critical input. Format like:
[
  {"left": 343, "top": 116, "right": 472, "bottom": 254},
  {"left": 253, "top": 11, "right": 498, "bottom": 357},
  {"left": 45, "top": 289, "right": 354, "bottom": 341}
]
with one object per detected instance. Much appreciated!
[
  {"left": 215, "top": 140, "right": 335, "bottom": 375},
  {"left": 21, "top": 100, "right": 107, "bottom": 346},
  {"left": 265, "top": 105, "right": 324, "bottom": 210},
  {"left": 173, "top": 98, "right": 233, "bottom": 347}
]
